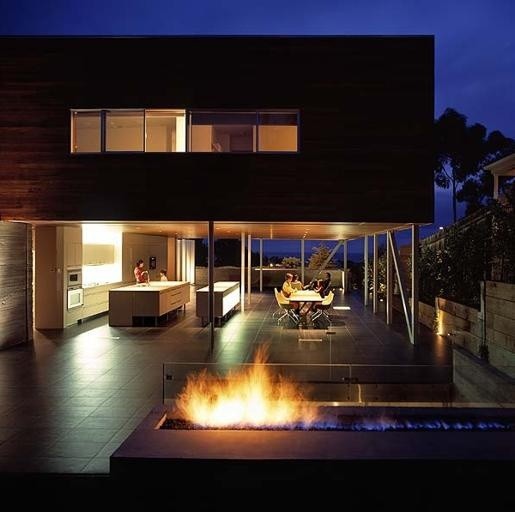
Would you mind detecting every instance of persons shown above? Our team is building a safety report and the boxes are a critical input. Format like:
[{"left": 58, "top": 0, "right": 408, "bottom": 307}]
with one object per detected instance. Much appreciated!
[
  {"left": 293, "top": 273, "right": 304, "bottom": 290},
  {"left": 159, "top": 268, "right": 168, "bottom": 281},
  {"left": 313, "top": 279, "right": 325, "bottom": 298},
  {"left": 323, "top": 272, "right": 331, "bottom": 289},
  {"left": 281, "top": 273, "right": 300, "bottom": 315},
  {"left": 133, "top": 259, "right": 145, "bottom": 283}
]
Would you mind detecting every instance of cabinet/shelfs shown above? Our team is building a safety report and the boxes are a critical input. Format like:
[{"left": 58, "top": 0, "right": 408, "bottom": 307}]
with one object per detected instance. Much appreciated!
[{"left": 159, "top": 282, "right": 190, "bottom": 317}]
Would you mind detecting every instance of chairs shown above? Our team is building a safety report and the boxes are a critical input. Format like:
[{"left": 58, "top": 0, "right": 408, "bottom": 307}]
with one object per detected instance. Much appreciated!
[
  {"left": 311, "top": 290, "right": 332, "bottom": 318},
  {"left": 274, "top": 288, "right": 298, "bottom": 325},
  {"left": 312, "top": 293, "right": 334, "bottom": 327},
  {"left": 272, "top": 290, "right": 296, "bottom": 316}
]
